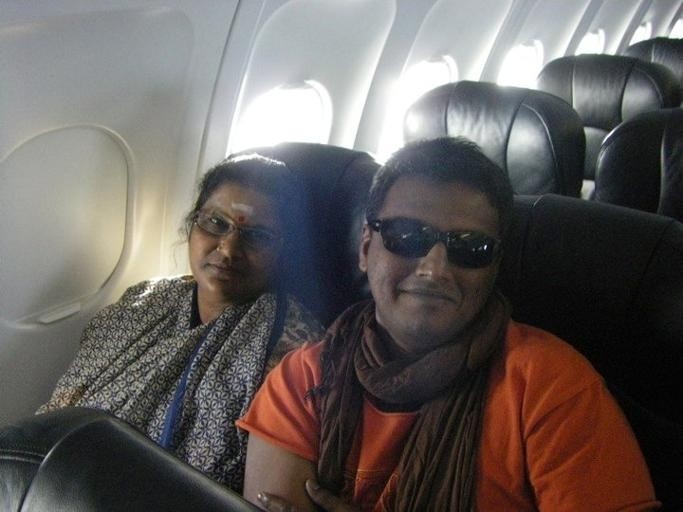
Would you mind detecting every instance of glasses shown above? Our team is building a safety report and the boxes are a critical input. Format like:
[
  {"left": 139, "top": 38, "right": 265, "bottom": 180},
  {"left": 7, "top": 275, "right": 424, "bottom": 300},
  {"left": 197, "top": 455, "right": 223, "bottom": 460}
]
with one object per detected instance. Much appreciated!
[
  {"left": 371, "top": 217, "right": 502, "bottom": 268},
  {"left": 192, "top": 209, "right": 277, "bottom": 250}
]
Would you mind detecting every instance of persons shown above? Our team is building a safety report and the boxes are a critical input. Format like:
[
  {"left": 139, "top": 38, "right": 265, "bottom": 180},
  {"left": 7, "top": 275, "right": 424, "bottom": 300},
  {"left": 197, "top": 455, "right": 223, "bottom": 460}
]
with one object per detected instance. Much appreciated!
[
  {"left": 32, "top": 153, "right": 327, "bottom": 495},
  {"left": 234, "top": 137, "right": 667, "bottom": 511}
]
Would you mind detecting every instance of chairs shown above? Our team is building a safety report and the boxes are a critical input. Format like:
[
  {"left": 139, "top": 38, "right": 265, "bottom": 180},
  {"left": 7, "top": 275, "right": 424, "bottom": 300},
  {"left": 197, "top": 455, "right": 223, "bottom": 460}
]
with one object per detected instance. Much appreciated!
[{"left": 0, "top": 33, "right": 683, "bottom": 511}]
